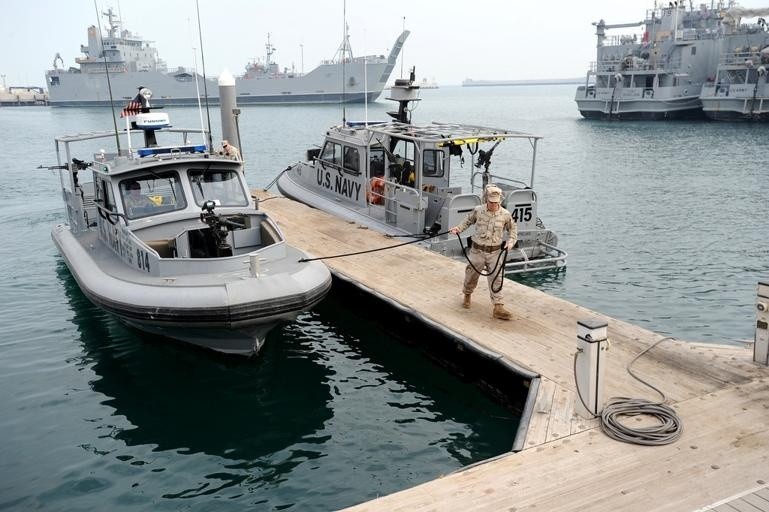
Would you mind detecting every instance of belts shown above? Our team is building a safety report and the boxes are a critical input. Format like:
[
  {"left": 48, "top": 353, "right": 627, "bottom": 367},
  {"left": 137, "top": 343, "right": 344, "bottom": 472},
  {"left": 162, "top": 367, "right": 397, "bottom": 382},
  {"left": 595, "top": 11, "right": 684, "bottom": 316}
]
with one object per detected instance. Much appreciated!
[{"left": 472, "top": 243, "right": 502, "bottom": 252}]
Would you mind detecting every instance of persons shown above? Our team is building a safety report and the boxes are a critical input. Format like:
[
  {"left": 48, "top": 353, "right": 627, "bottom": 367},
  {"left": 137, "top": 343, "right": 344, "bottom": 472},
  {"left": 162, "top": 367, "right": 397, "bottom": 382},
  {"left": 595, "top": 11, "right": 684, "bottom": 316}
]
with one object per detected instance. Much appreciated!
[
  {"left": 221, "top": 139, "right": 244, "bottom": 172},
  {"left": 123, "top": 181, "right": 154, "bottom": 211},
  {"left": 448, "top": 186, "right": 518, "bottom": 320}
]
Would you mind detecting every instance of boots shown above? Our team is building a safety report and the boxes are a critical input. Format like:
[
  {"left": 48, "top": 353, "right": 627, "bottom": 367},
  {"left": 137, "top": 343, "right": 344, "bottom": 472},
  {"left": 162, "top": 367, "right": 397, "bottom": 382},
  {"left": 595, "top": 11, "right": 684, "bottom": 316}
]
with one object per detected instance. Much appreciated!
[
  {"left": 463, "top": 294, "right": 471, "bottom": 308},
  {"left": 493, "top": 304, "right": 513, "bottom": 319}
]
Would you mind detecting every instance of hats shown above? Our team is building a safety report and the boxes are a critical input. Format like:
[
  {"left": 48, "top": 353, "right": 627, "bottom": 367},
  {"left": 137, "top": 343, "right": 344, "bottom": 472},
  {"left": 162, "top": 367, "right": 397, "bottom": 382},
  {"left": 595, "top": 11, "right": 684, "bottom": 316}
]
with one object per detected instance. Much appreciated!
[
  {"left": 486, "top": 187, "right": 502, "bottom": 203},
  {"left": 221, "top": 140, "right": 227, "bottom": 147}
]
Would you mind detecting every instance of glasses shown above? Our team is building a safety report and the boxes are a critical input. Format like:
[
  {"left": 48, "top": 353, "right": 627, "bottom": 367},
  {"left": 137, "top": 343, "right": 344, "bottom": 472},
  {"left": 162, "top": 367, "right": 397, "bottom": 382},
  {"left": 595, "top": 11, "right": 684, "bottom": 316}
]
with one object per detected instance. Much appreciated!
[{"left": 131, "top": 187, "right": 141, "bottom": 190}]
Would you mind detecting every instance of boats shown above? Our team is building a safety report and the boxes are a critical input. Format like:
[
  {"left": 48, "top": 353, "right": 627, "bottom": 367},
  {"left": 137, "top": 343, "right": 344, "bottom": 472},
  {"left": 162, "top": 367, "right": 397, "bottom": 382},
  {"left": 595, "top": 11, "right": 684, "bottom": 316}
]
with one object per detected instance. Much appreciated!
[
  {"left": 35, "top": 5, "right": 334, "bottom": 362},
  {"left": 696, "top": 44, "right": 769, "bottom": 123},
  {"left": 572, "top": 0, "right": 769, "bottom": 122},
  {"left": 41, "top": 250, "right": 333, "bottom": 459},
  {"left": 274, "top": 3, "right": 569, "bottom": 278},
  {"left": 43, "top": 5, "right": 412, "bottom": 104}
]
